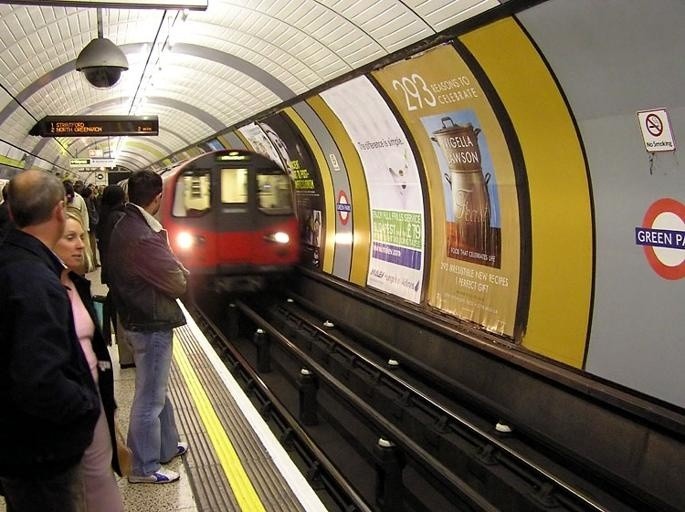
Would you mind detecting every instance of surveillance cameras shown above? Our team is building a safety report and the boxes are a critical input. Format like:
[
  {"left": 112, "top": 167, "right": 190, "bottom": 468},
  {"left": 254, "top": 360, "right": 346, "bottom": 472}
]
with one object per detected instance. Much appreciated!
[{"left": 75, "top": 38, "right": 131, "bottom": 88}]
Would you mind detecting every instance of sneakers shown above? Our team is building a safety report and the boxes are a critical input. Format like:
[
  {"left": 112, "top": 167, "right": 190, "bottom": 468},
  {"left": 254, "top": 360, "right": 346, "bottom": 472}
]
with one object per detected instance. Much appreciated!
[
  {"left": 160, "top": 441, "right": 188, "bottom": 465},
  {"left": 128, "top": 464, "right": 181, "bottom": 484}
]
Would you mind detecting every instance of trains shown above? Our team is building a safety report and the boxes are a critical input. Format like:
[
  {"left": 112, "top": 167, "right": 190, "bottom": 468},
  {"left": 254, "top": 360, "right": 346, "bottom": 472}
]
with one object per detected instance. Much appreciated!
[{"left": 115, "top": 148, "right": 303, "bottom": 295}]
